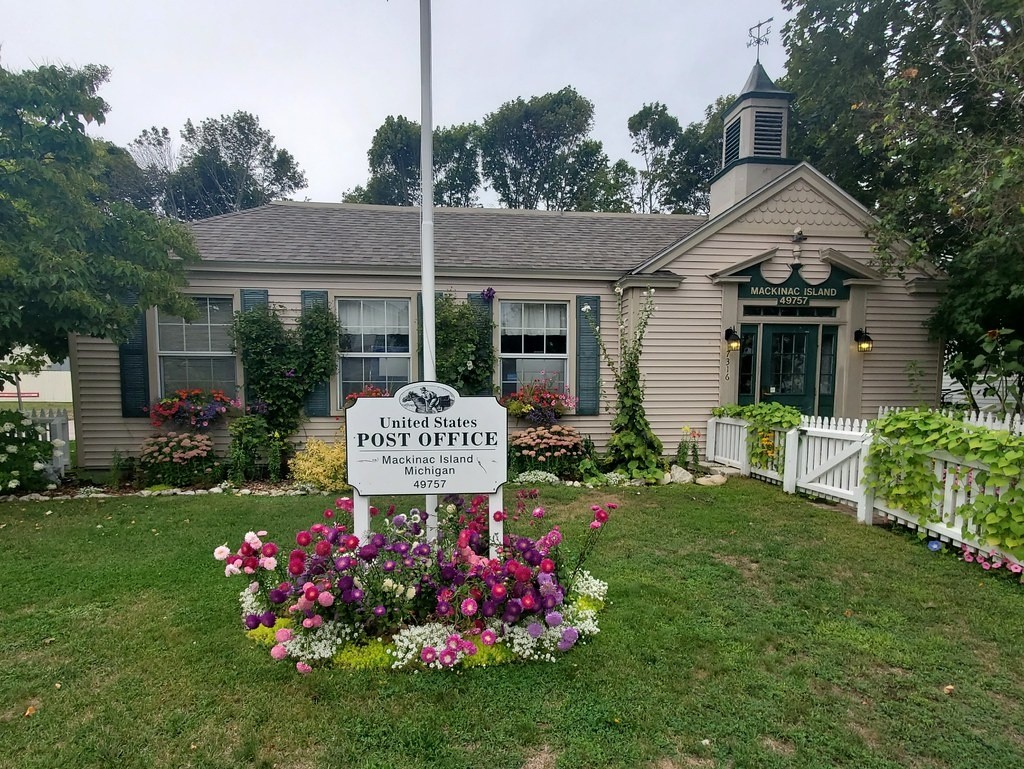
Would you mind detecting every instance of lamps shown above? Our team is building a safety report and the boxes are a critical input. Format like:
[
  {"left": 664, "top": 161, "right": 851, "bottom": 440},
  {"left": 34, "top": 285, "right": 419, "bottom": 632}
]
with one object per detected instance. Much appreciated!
[
  {"left": 725, "top": 328, "right": 740, "bottom": 352},
  {"left": 854, "top": 325, "right": 873, "bottom": 353}
]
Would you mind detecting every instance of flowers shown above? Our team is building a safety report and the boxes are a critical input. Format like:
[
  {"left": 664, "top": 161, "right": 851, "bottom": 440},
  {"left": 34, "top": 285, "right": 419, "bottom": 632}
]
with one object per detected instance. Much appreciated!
[
  {"left": 343, "top": 385, "right": 391, "bottom": 408},
  {"left": 504, "top": 370, "right": 578, "bottom": 425},
  {"left": 145, "top": 389, "right": 241, "bottom": 431}
]
